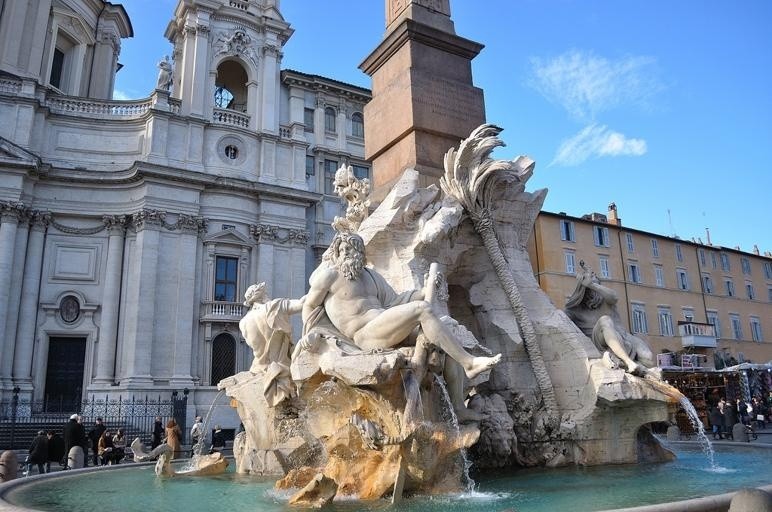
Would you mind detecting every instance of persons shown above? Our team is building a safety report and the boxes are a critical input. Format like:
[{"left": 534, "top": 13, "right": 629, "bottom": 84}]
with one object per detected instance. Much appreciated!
[
  {"left": 112, "top": 427, "right": 127, "bottom": 463},
  {"left": 301, "top": 229, "right": 502, "bottom": 380},
  {"left": 165, "top": 421, "right": 181, "bottom": 459},
  {"left": 150, "top": 416, "right": 165, "bottom": 462},
  {"left": 88, "top": 418, "right": 106, "bottom": 465},
  {"left": 239, "top": 282, "right": 309, "bottom": 371},
  {"left": 26, "top": 413, "right": 89, "bottom": 477},
  {"left": 98, "top": 427, "right": 114, "bottom": 467},
  {"left": 563, "top": 259, "right": 660, "bottom": 382},
  {"left": 710, "top": 391, "right": 772, "bottom": 440},
  {"left": 190, "top": 416, "right": 208, "bottom": 458},
  {"left": 212, "top": 424, "right": 225, "bottom": 447}
]
